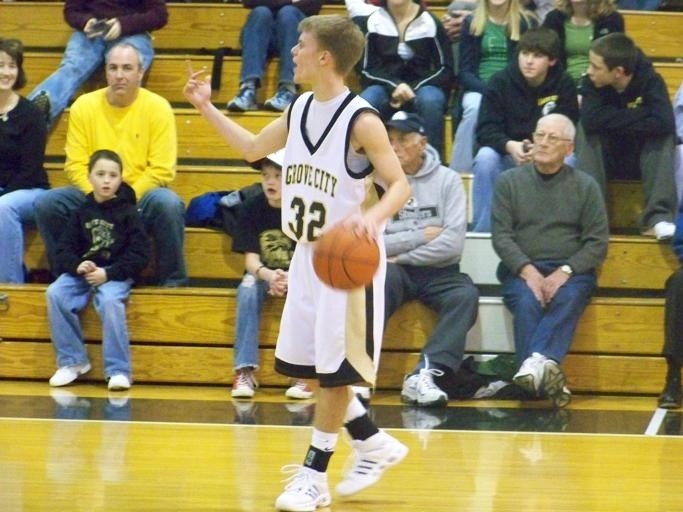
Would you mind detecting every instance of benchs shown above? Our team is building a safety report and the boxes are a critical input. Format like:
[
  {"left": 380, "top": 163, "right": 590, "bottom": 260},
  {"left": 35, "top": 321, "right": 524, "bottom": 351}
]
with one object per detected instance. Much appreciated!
[{"left": 0, "top": 1, "right": 683, "bottom": 397}]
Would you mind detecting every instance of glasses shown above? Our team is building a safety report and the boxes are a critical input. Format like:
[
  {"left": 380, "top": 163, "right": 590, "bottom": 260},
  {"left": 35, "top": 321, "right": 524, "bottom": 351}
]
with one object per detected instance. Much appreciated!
[{"left": 532, "top": 131, "right": 572, "bottom": 144}]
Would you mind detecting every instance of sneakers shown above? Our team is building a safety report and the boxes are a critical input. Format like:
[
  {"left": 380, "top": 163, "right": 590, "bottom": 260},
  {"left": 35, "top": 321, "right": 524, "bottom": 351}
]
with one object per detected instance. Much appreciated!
[
  {"left": 285, "top": 380, "right": 316, "bottom": 400},
  {"left": 656, "top": 378, "right": 683, "bottom": 409},
  {"left": 263, "top": 87, "right": 298, "bottom": 113},
  {"left": 230, "top": 367, "right": 259, "bottom": 399},
  {"left": 641, "top": 220, "right": 677, "bottom": 242},
  {"left": 399, "top": 353, "right": 449, "bottom": 409},
  {"left": 336, "top": 427, "right": 410, "bottom": 497},
  {"left": 48, "top": 359, "right": 92, "bottom": 387},
  {"left": 511, "top": 351, "right": 573, "bottom": 410},
  {"left": 274, "top": 463, "right": 332, "bottom": 512},
  {"left": 350, "top": 384, "right": 372, "bottom": 403},
  {"left": 29, "top": 88, "right": 52, "bottom": 132},
  {"left": 227, "top": 87, "right": 259, "bottom": 112},
  {"left": 107, "top": 375, "right": 131, "bottom": 390}
]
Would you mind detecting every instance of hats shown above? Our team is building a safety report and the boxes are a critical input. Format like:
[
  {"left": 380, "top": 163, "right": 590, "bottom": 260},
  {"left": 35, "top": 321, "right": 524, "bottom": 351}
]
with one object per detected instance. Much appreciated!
[
  {"left": 244, "top": 147, "right": 287, "bottom": 170},
  {"left": 385, "top": 110, "right": 427, "bottom": 136}
]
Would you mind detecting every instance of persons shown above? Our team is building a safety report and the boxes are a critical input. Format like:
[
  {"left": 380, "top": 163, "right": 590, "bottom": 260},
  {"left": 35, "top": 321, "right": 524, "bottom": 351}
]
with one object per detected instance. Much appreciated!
[
  {"left": 49, "top": 391, "right": 133, "bottom": 420},
  {"left": 25, "top": 0, "right": 169, "bottom": 130},
  {"left": 0, "top": 39, "right": 62, "bottom": 283},
  {"left": 185, "top": 13, "right": 410, "bottom": 511},
  {"left": 47, "top": 148, "right": 150, "bottom": 389},
  {"left": 33, "top": 41, "right": 188, "bottom": 288},
  {"left": 226, "top": 1, "right": 683, "bottom": 412}
]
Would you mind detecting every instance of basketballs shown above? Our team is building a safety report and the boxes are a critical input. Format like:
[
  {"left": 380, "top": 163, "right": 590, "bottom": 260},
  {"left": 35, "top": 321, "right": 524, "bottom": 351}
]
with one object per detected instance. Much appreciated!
[{"left": 313, "top": 225, "right": 379, "bottom": 289}]
